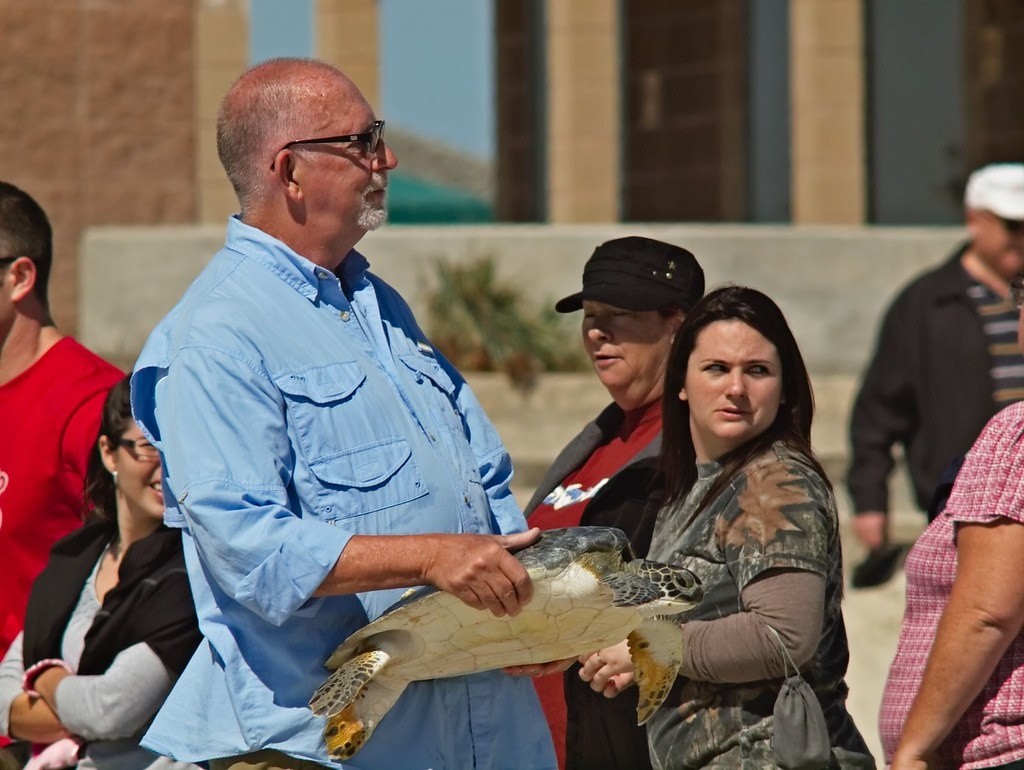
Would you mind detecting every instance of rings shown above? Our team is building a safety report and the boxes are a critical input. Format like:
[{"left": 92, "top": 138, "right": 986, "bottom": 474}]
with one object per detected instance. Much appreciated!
[{"left": 597, "top": 652, "right": 605, "bottom": 664}]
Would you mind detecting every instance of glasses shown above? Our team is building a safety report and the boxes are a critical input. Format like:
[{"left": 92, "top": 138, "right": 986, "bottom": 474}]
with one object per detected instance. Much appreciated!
[
  {"left": 119, "top": 436, "right": 160, "bottom": 457},
  {"left": 269, "top": 119, "right": 386, "bottom": 171}
]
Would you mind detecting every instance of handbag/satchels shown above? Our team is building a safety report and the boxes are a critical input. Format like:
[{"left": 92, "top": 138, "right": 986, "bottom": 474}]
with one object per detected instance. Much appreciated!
[{"left": 765, "top": 622, "right": 832, "bottom": 770}]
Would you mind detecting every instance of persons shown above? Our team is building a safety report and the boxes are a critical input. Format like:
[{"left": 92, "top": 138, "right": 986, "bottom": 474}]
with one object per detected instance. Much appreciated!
[
  {"left": 0, "top": 181, "right": 206, "bottom": 770},
  {"left": 131, "top": 58, "right": 579, "bottom": 770},
  {"left": 880, "top": 280, "right": 1024, "bottom": 770},
  {"left": 844, "top": 165, "right": 1024, "bottom": 550},
  {"left": 523, "top": 236, "right": 876, "bottom": 770}
]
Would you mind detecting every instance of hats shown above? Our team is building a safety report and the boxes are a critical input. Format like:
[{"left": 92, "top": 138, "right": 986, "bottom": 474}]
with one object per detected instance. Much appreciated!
[
  {"left": 555, "top": 235, "right": 705, "bottom": 312},
  {"left": 964, "top": 162, "right": 1024, "bottom": 222}
]
